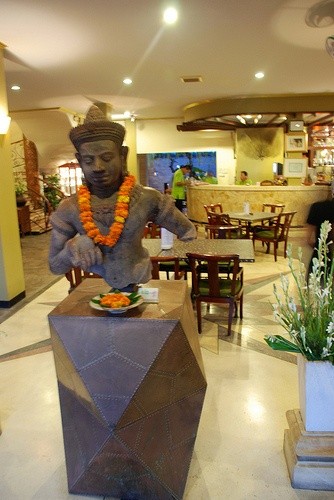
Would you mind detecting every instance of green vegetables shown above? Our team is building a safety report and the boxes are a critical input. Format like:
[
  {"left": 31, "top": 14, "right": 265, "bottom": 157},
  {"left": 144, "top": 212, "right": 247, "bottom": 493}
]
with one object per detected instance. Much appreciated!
[{"left": 91, "top": 289, "right": 141, "bottom": 306}]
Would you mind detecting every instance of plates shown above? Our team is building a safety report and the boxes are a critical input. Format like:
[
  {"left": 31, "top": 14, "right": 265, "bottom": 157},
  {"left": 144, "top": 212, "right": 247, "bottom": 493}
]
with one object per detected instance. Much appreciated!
[{"left": 88, "top": 291, "right": 144, "bottom": 314}]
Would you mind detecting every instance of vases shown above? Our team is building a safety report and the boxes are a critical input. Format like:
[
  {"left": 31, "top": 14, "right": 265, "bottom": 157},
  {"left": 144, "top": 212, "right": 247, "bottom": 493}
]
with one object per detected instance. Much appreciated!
[{"left": 296, "top": 352, "right": 334, "bottom": 433}]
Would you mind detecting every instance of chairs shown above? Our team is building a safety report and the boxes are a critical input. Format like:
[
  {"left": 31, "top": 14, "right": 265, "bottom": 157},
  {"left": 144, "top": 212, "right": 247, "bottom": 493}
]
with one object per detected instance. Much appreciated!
[
  {"left": 204, "top": 203, "right": 298, "bottom": 262},
  {"left": 184, "top": 252, "right": 244, "bottom": 337}
]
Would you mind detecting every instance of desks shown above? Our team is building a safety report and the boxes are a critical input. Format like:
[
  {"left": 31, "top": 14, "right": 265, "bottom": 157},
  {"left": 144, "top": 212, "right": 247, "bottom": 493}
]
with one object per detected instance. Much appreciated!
[
  {"left": 47, "top": 272, "right": 207, "bottom": 500},
  {"left": 74, "top": 239, "right": 163, "bottom": 286},
  {"left": 156, "top": 239, "right": 255, "bottom": 318},
  {"left": 228, "top": 211, "right": 278, "bottom": 239},
  {"left": 185, "top": 186, "right": 329, "bottom": 227}
]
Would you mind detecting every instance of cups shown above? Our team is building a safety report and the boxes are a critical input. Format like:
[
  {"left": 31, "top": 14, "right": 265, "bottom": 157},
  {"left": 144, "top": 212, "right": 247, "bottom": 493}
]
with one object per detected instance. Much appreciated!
[
  {"left": 161, "top": 228, "right": 174, "bottom": 250},
  {"left": 244, "top": 203, "right": 249, "bottom": 215}
]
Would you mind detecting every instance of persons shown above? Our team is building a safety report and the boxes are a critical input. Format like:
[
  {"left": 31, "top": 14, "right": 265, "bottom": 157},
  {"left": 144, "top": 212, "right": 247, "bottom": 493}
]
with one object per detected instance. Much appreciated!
[
  {"left": 204, "top": 172, "right": 218, "bottom": 184},
  {"left": 171, "top": 164, "right": 191, "bottom": 212},
  {"left": 48, "top": 103, "right": 197, "bottom": 287},
  {"left": 236, "top": 171, "right": 253, "bottom": 184}
]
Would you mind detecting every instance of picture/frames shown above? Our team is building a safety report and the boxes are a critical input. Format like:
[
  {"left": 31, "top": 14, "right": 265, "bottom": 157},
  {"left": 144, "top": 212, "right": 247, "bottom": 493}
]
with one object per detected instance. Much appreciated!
[
  {"left": 283, "top": 158, "right": 308, "bottom": 179},
  {"left": 284, "top": 132, "right": 308, "bottom": 154}
]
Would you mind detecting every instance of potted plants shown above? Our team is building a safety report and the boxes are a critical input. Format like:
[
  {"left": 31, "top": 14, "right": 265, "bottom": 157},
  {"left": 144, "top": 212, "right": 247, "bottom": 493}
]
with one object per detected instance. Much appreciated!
[{"left": 13, "top": 181, "right": 28, "bottom": 207}]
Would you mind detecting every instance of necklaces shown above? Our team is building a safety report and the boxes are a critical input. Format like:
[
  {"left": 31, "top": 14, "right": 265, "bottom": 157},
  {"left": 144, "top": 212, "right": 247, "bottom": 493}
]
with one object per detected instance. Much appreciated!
[{"left": 78, "top": 173, "right": 135, "bottom": 246}]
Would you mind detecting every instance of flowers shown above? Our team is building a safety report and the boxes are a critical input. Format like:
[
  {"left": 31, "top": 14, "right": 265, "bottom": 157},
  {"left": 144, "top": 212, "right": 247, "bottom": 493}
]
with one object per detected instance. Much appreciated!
[{"left": 263, "top": 219, "right": 334, "bottom": 365}]
